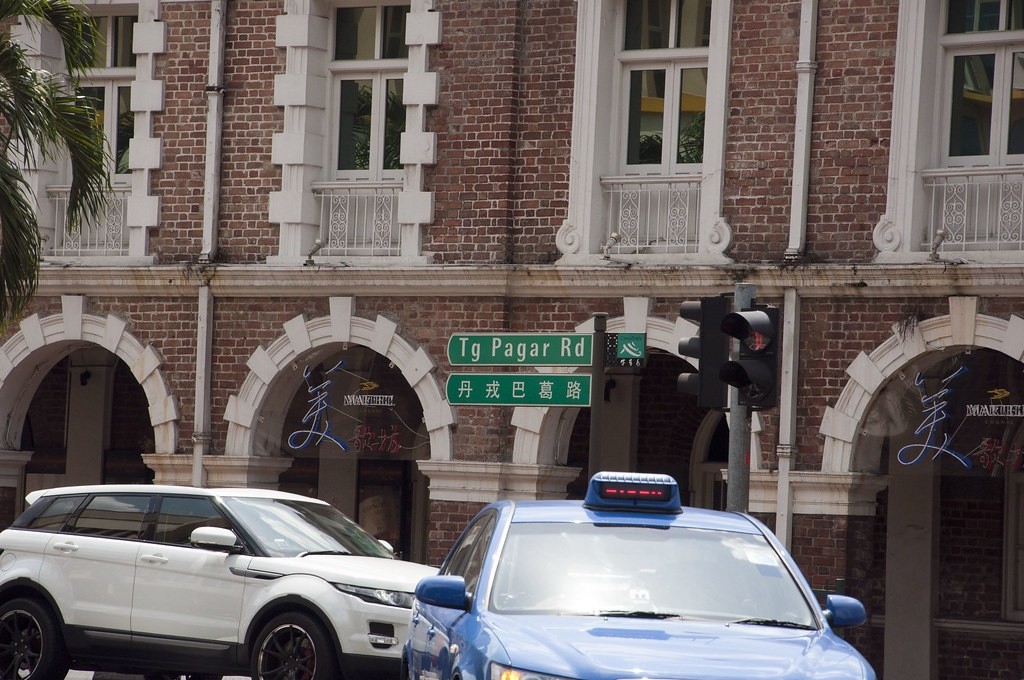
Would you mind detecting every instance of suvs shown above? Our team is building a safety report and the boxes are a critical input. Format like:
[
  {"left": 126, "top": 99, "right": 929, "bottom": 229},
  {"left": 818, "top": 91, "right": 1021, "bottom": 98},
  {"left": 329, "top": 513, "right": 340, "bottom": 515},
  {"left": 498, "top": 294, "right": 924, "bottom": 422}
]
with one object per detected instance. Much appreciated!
[{"left": 0, "top": 483, "right": 444, "bottom": 680}]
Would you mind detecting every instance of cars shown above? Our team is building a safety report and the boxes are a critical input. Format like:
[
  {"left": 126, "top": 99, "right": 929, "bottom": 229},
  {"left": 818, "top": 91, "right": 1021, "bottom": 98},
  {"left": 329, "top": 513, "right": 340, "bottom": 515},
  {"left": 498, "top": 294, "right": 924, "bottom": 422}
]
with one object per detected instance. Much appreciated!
[{"left": 400, "top": 471, "right": 880, "bottom": 680}]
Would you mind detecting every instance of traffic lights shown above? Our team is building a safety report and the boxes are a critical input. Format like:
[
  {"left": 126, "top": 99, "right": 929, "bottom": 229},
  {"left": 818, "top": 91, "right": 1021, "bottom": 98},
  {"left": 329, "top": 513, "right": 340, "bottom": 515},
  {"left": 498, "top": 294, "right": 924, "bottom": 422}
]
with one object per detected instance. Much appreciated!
[{"left": 720, "top": 302, "right": 782, "bottom": 409}]
